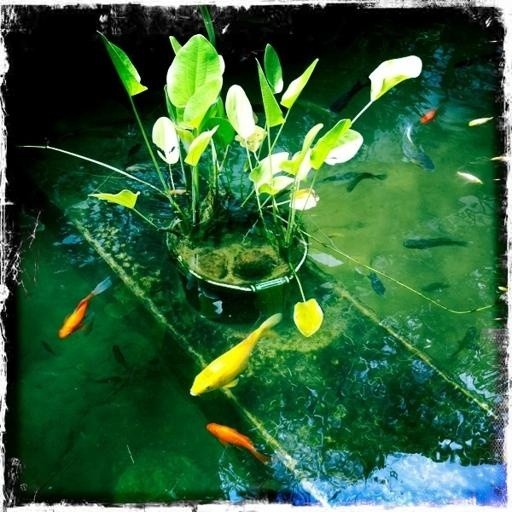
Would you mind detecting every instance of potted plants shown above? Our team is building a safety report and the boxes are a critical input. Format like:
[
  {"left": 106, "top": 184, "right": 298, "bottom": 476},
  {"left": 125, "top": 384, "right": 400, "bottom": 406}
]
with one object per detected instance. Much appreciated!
[{"left": 86, "top": 6, "right": 424, "bottom": 338}]
